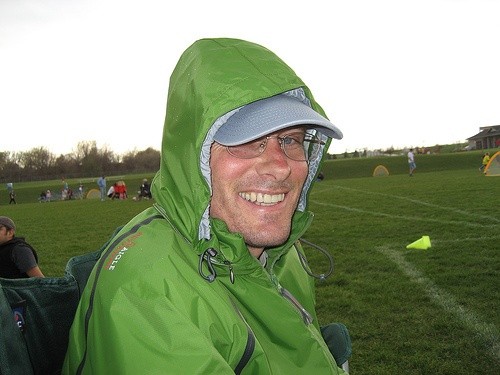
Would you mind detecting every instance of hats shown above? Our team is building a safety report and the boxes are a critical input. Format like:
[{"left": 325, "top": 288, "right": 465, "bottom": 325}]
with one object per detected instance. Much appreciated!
[
  {"left": 0, "top": 216, "right": 17, "bottom": 232},
  {"left": 212, "top": 94, "right": 344, "bottom": 147}
]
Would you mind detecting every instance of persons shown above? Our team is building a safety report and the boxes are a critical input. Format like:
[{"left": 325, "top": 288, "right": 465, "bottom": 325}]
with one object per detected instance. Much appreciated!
[
  {"left": 406, "top": 149, "right": 417, "bottom": 177},
  {"left": 10, "top": 191, "right": 17, "bottom": 205},
  {"left": 61, "top": 37, "right": 350, "bottom": 375},
  {"left": 7, "top": 181, "right": 13, "bottom": 195},
  {"left": 40, "top": 190, "right": 51, "bottom": 203},
  {"left": 478, "top": 153, "right": 490, "bottom": 173},
  {"left": 0, "top": 216, "right": 46, "bottom": 280}
]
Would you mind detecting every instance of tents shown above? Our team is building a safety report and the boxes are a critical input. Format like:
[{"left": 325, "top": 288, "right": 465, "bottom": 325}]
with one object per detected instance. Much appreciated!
[
  {"left": 484, "top": 152, "right": 500, "bottom": 177},
  {"left": 373, "top": 165, "right": 389, "bottom": 177}
]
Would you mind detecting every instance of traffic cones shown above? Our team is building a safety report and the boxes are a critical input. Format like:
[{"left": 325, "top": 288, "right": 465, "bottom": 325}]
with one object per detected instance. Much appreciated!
[{"left": 406, "top": 234, "right": 433, "bottom": 250}]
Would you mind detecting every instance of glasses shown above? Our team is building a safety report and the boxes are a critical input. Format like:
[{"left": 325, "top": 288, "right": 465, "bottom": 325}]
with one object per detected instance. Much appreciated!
[{"left": 226, "top": 129, "right": 326, "bottom": 162}]
